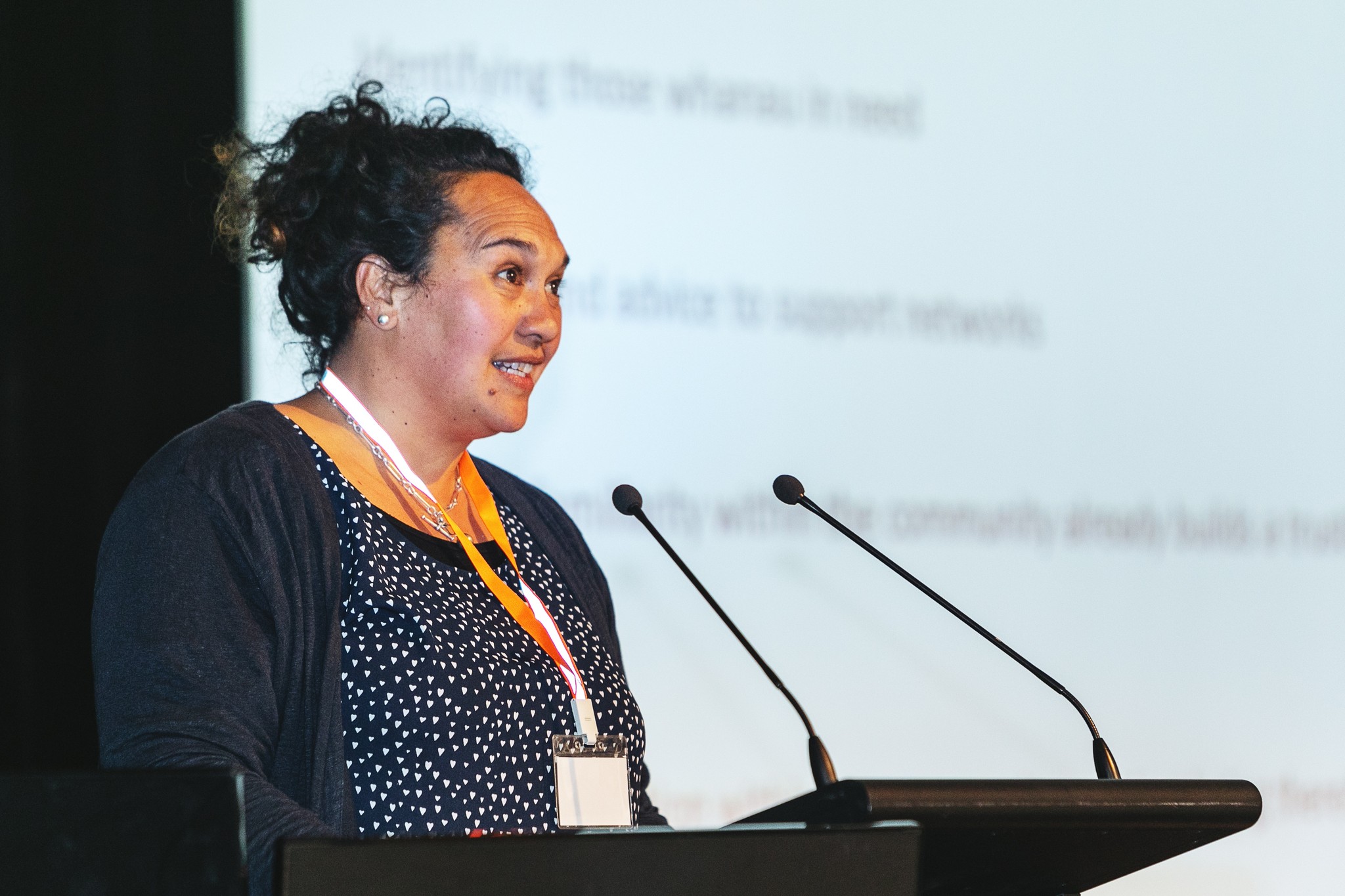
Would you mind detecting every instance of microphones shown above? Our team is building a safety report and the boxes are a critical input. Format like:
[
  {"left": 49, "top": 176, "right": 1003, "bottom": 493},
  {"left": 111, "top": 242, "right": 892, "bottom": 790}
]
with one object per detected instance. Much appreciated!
[
  {"left": 773, "top": 475, "right": 1121, "bottom": 780},
  {"left": 612, "top": 484, "right": 835, "bottom": 790}
]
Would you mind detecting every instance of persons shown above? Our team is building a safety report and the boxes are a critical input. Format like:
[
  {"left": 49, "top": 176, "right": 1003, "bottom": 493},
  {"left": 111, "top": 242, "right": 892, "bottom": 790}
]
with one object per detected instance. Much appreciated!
[{"left": 89, "top": 81, "right": 666, "bottom": 896}]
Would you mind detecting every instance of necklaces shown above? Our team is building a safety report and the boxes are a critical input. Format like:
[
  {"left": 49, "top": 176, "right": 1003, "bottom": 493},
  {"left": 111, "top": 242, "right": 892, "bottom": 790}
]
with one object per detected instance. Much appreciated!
[{"left": 314, "top": 383, "right": 473, "bottom": 546}]
